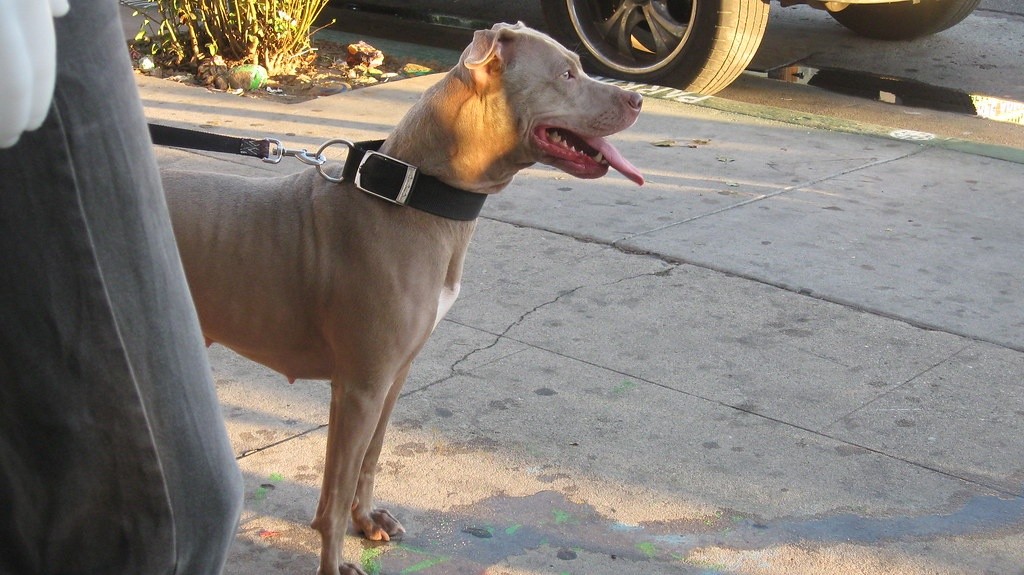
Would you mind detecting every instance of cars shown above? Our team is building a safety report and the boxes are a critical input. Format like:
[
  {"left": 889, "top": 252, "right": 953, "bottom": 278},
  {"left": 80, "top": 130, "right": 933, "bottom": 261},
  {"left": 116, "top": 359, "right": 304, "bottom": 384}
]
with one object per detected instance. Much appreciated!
[{"left": 281, "top": 0, "right": 984, "bottom": 99}]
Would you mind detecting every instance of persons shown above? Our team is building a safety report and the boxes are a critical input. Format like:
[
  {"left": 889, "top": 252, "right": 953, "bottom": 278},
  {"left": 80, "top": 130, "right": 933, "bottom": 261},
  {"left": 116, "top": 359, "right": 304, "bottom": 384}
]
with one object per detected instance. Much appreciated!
[{"left": 1, "top": 0, "right": 246, "bottom": 570}]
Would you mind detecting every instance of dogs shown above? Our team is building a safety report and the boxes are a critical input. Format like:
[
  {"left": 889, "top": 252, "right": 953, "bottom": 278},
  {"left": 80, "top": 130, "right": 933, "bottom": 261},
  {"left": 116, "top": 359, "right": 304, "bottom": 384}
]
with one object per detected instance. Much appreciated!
[{"left": 159, "top": 22, "right": 645, "bottom": 575}]
